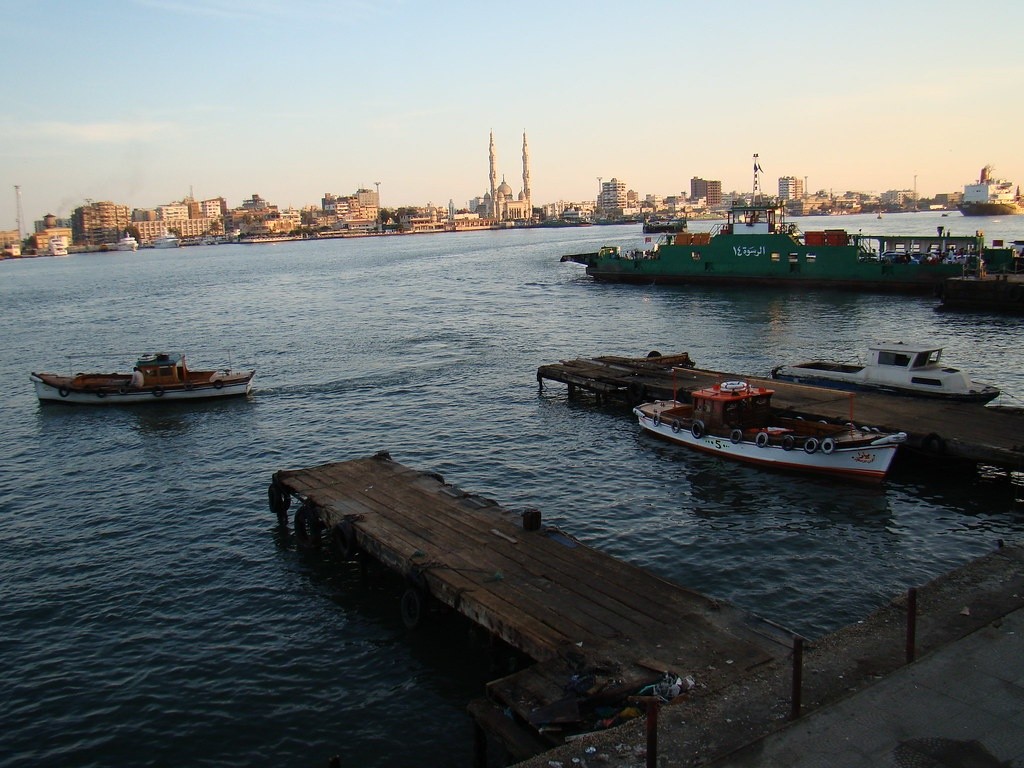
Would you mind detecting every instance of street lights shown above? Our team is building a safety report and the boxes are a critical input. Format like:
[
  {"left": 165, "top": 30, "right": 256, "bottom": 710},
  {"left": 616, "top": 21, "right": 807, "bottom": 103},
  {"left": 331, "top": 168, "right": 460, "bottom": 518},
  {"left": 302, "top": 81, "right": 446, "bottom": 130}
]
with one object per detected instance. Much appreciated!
[
  {"left": 373, "top": 181, "right": 382, "bottom": 224},
  {"left": 84, "top": 198, "right": 94, "bottom": 245}
]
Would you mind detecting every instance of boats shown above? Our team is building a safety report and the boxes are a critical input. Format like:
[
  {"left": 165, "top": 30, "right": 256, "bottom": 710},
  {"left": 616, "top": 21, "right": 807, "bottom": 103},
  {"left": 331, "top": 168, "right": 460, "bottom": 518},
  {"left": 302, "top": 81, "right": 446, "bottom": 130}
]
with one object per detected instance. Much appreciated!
[
  {"left": 116, "top": 231, "right": 139, "bottom": 251},
  {"left": 558, "top": 151, "right": 1024, "bottom": 288},
  {"left": 150, "top": 226, "right": 182, "bottom": 248},
  {"left": 642, "top": 203, "right": 688, "bottom": 235},
  {"left": 46, "top": 236, "right": 68, "bottom": 256},
  {"left": 956, "top": 161, "right": 1024, "bottom": 216},
  {"left": 772, "top": 340, "right": 1003, "bottom": 406},
  {"left": 631, "top": 368, "right": 908, "bottom": 484},
  {"left": 938, "top": 232, "right": 1024, "bottom": 312},
  {"left": 26, "top": 350, "right": 257, "bottom": 407}
]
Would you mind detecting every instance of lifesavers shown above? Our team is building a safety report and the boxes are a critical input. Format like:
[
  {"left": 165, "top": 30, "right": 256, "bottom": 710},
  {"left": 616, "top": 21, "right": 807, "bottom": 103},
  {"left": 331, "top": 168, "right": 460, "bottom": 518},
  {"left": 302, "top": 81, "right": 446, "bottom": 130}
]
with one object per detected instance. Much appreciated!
[
  {"left": 214, "top": 379, "right": 223, "bottom": 389},
  {"left": 755, "top": 432, "right": 768, "bottom": 448},
  {"left": 821, "top": 438, "right": 835, "bottom": 455},
  {"left": 729, "top": 429, "right": 743, "bottom": 444},
  {"left": 804, "top": 438, "right": 819, "bottom": 455},
  {"left": 139, "top": 353, "right": 157, "bottom": 362},
  {"left": 400, "top": 586, "right": 424, "bottom": 630},
  {"left": 118, "top": 386, "right": 127, "bottom": 395},
  {"left": 796, "top": 415, "right": 879, "bottom": 434},
  {"left": 59, "top": 385, "right": 69, "bottom": 397},
  {"left": 781, "top": 435, "right": 795, "bottom": 451},
  {"left": 720, "top": 381, "right": 747, "bottom": 392},
  {"left": 653, "top": 413, "right": 660, "bottom": 427},
  {"left": 152, "top": 385, "right": 164, "bottom": 397},
  {"left": 97, "top": 388, "right": 106, "bottom": 398},
  {"left": 691, "top": 420, "right": 705, "bottom": 439},
  {"left": 267, "top": 481, "right": 291, "bottom": 514},
  {"left": 671, "top": 419, "right": 680, "bottom": 434},
  {"left": 333, "top": 520, "right": 355, "bottom": 556},
  {"left": 294, "top": 506, "right": 321, "bottom": 549}
]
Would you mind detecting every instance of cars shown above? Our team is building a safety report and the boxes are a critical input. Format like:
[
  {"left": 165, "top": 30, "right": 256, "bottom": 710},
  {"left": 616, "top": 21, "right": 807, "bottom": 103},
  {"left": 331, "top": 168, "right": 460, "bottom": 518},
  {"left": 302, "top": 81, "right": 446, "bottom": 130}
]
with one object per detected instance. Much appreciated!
[{"left": 858, "top": 245, "right": 985, "bottom": 265}]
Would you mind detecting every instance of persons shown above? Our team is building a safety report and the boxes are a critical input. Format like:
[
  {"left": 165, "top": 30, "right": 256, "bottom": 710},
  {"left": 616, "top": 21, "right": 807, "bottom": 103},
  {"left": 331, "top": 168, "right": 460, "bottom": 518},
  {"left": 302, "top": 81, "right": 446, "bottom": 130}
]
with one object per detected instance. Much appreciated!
[
  {"left": 898, "top": 244, "right": 987, "bottom": 265},
  {"left": 756, "top": 396, "right": 768, "bottom": 413},
  {"left": 750, "top": 215, "right": 759, "bottom": 222},
  {"left": 645, "top": 250, "right": 659, "bottom": 259},
  {"left": 625, "top": 251, "right": 634, "bottom": 259},
  {"left": 129, "top": 367, "right": 144, "bottom": 387}
]
